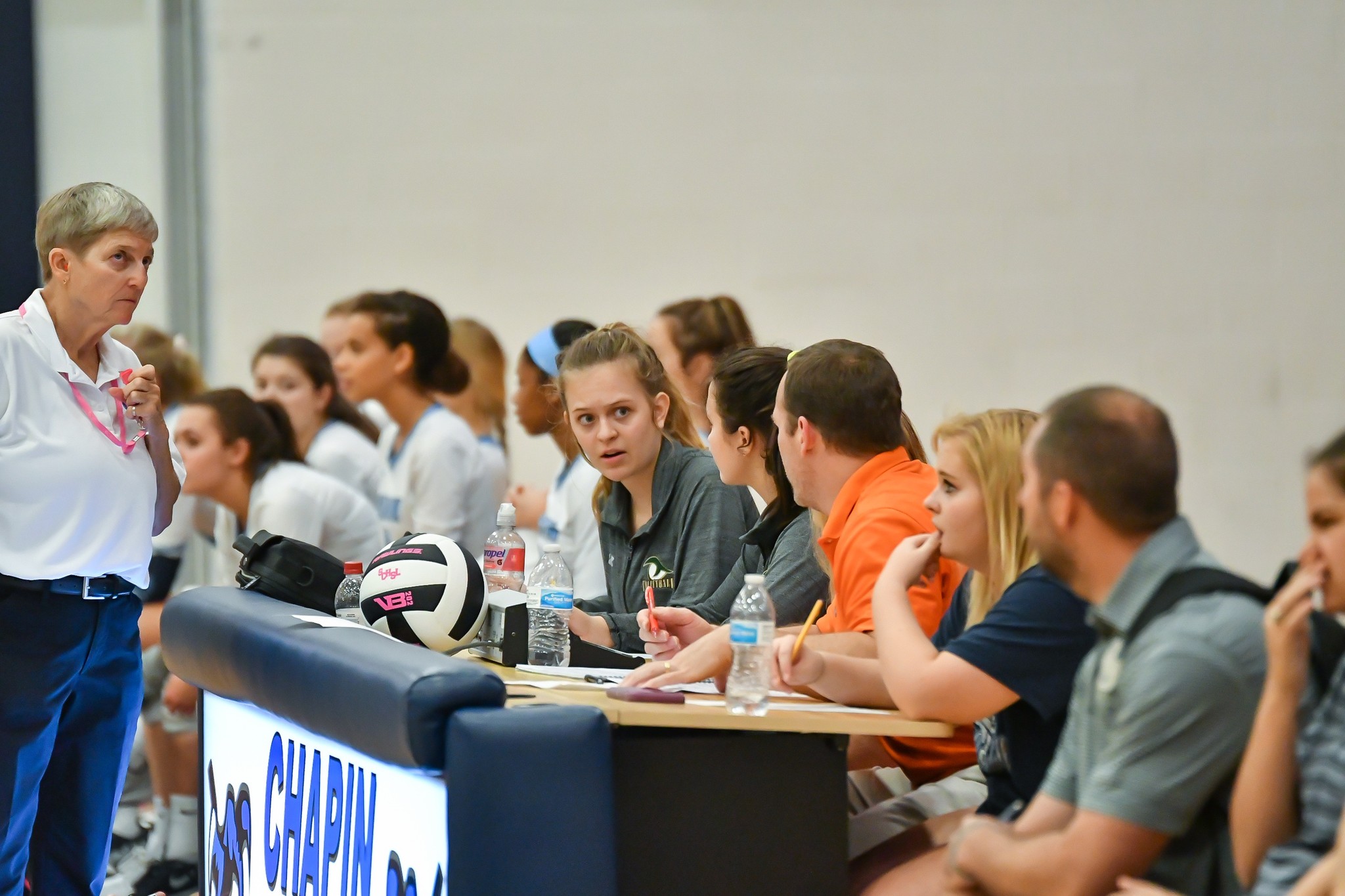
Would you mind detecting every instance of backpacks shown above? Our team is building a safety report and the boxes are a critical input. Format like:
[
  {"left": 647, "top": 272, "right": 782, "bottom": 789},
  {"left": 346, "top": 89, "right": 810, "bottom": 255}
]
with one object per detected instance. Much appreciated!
[
  {"left": 231, "top": 530, "right": 345, "bottom": 617},
  {"left": 1113, "top": 558, "right": 1344, "bottom": 704}
]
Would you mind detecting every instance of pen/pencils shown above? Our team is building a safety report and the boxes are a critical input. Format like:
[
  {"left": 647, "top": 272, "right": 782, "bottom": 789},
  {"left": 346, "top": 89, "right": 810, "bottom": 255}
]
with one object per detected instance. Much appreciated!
[
  {"left": 584, "top": 674, "right": 603, "bottom": 684},
  {"left": 645, "top": 585, "right": 657, "bottom": 640},
  {"left": 792, "top": 599, "right": 824, "bottom": 660}
]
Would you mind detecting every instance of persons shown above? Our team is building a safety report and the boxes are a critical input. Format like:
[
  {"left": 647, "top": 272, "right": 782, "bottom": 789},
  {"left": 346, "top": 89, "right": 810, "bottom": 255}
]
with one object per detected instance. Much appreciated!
[
  {"left": 111, "top": 289, "right": 930, "bottom": 896},
  {"left": 1114, "top": 432, "right": 1345, "bottom": 894},
  {"left": 771, "top": 407, "right": 1098, "bottom": 896},
  {"left": 940, "top": 386, "right": 1317, "bottom": 896},
  {"left": 0, "top": 182, "right": 188, "bottom": 896},
  {"left": 621, "top": 339, "right": 989, "bottom": 863}
]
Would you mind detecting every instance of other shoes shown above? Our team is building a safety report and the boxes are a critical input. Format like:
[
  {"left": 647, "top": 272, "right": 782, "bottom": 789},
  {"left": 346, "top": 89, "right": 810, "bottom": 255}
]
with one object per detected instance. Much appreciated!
[
  {"left": 98, "top": 845, "right": 153, "bottom": 896},
  {"left": 104, "top": 826, "right": 148, "bottom": 875},
  {"left": 128, "top": 859, "right": 198, "bottom": 896}
]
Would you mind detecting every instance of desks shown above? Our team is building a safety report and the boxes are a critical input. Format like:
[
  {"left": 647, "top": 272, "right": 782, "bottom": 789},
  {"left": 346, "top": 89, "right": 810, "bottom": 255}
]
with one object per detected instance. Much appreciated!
[{"left": 155, "top": 591, "right": 959, "bottom": 896}]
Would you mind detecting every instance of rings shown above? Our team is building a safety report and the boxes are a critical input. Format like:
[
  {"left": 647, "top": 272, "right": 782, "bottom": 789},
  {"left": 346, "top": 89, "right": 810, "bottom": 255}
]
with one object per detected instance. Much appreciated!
[
  {"left": 664, "top": 661, "right": 671, "bottom": 673},
  {"left": 132, "top": 405, "right": 136, "bottom": 417}
]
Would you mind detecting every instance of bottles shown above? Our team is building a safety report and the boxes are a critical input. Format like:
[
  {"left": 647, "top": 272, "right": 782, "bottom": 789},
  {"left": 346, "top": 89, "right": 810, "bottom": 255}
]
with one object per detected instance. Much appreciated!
[
  {"left": 482, "top": 502, "right": 526, "bottom": 594},
  {"left": 526, "top": 544, "right": 573, "bottom": 668},
  {"left": 723, "top": 573, "right": 778, "bottom": 716},
  {"left": 334, "top": 560, "right": 364, "bottom": 627}
]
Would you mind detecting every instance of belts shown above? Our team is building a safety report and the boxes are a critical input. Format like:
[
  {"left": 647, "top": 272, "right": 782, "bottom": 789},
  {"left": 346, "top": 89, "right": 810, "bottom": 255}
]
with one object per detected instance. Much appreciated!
[{"left": 0, "top": 574, "right": 137, "bottom": 600}]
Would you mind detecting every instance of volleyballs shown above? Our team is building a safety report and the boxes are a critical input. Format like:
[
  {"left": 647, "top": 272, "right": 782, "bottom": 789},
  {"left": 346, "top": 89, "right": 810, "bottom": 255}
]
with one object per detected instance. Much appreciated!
[{"left": 359, "top": 534, "right": 488, "bottom": 657}]
